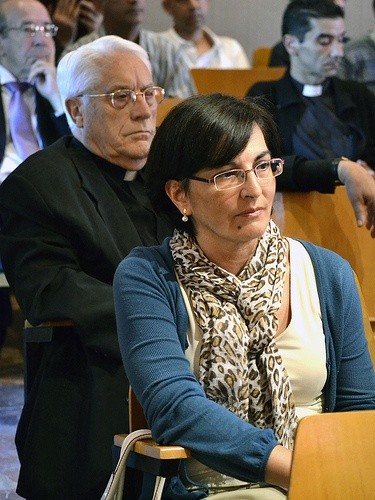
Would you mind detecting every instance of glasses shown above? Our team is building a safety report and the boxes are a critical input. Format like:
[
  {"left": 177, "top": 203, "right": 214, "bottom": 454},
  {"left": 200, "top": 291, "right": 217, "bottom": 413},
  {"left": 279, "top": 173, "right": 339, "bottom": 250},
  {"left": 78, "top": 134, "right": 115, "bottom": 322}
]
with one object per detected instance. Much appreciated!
[
  {"left": 77, "top": 85, "right": 165, "bottom": 110},
  {"left": 190, "top": 157, "right": 284, "bottom": 191},
  {"left": 6, "top": 23, "right": 58, "bottom": 37}
]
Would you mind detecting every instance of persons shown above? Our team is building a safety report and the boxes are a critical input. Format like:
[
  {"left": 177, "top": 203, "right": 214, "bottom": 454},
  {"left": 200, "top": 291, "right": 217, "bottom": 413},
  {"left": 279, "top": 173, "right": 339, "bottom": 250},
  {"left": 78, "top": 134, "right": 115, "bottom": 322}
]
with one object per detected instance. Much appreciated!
[
  {"left": 0, "top": 34, "right": 375, "bottom": 500},
  {"left": 0, "top": 0, "right": 71, "bottom": 183},
  {"left": 57, "top": 0, "right": 197, "bottom": 99},
  {"left": 162, "top": 0, "right": 252, "bottom": 70},
  {"left": 113, "top": 93, "right": 375, "bottom": 500},
  {"left": 245, "top": 0, "right": 375, "bottom": 175}
]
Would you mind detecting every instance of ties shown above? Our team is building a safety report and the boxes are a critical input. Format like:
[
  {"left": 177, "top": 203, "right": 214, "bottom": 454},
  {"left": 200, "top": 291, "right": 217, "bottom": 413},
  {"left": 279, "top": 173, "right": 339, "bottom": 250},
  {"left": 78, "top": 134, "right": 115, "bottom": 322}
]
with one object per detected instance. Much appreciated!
[{"left": 3, "top": 82, "right": 39, "bottom": 160}]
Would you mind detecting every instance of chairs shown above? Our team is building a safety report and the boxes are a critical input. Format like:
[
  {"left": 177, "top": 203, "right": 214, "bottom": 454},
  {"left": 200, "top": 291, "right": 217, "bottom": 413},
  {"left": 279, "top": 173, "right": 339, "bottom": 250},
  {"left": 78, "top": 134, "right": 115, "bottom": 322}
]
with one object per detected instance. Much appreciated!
[
  {"left": 156, "top": 46, "right": 375, "bottom": 323},
  {"left": 111, "top": 269, "right": 375, "bottom": 500}
]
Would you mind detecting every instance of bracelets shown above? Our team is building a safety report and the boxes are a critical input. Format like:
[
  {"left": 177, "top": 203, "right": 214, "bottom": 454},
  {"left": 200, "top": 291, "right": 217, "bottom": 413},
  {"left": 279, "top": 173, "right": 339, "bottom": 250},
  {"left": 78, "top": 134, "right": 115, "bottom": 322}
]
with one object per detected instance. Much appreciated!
[{"left": 331, "top": 156, "right": 347, "bottom": 186}]
